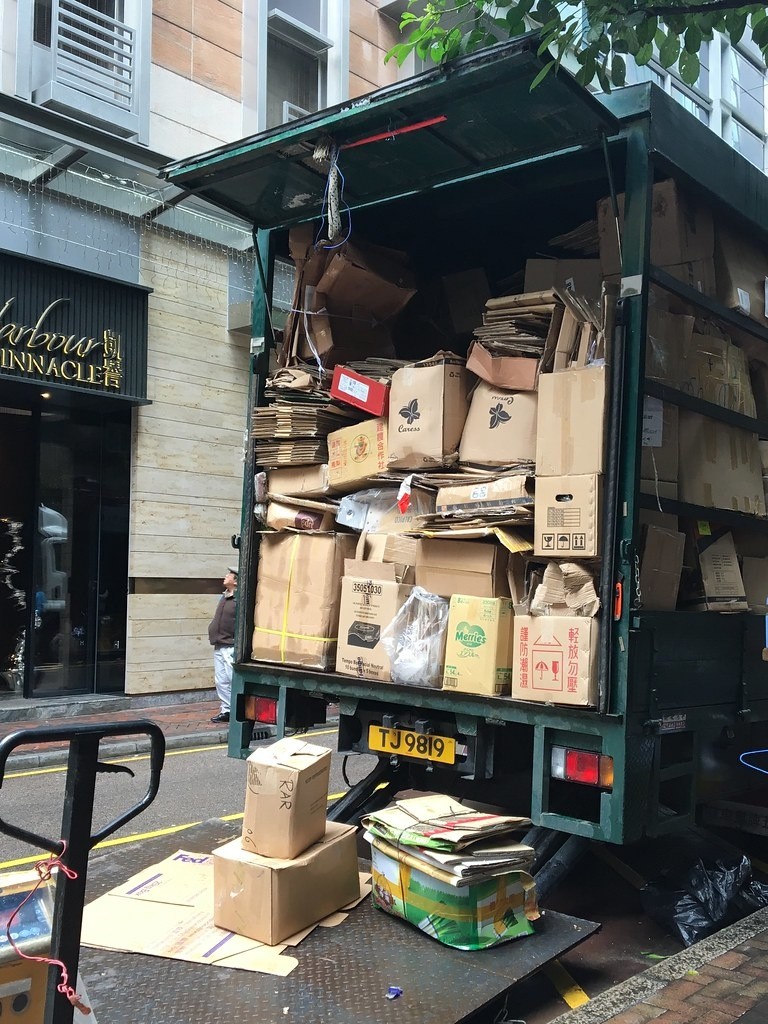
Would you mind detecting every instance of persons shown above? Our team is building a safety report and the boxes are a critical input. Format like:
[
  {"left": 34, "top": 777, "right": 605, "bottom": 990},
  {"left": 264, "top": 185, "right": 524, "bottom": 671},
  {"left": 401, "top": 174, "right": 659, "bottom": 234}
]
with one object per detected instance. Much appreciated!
[
  {"left": 207, "top": 566, "right": 239, "bottom": 724},
  {"left": 35, "top": 586, "right": 47, "bottom": 615}
]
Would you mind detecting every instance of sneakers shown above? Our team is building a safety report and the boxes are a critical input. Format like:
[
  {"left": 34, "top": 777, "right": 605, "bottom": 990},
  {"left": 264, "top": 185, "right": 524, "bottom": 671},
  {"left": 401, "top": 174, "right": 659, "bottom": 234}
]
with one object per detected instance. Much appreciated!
[{"left": 211, "top": 712, "right": 230, "bottom": 723}]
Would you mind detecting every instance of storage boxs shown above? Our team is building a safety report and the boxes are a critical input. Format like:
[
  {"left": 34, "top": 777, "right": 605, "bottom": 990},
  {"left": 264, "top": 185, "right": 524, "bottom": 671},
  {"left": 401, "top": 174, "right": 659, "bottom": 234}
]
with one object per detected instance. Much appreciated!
[
  {"left": 370, "top": 844, "right": 542, "bottom": 950},
  {"left": 0, "top": 867, "right": 55, "bottom": 1024},
  {"left": 249, "top": 174, "right": 768, "bottom": 712},
  {"left": 241, "top": 736, "right": 333, "bottom": 862},
  {"left": 211, "top": 819, "right": 361, "bottom": 947}
]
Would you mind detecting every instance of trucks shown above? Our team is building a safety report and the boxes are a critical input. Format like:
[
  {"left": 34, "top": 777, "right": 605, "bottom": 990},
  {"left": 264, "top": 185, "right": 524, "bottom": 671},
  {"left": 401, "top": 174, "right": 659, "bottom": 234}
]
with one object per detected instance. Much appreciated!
[{"left": 159, "top": 22, "right": 768, "bottom": 848}]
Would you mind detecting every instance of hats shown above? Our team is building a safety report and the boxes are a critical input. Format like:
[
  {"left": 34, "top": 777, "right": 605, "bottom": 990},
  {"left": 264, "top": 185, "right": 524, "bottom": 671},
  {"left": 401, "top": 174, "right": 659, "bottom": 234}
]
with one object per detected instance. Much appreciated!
[{"left": 228, "top": 565, "right": 238, "bottom": 574}]
